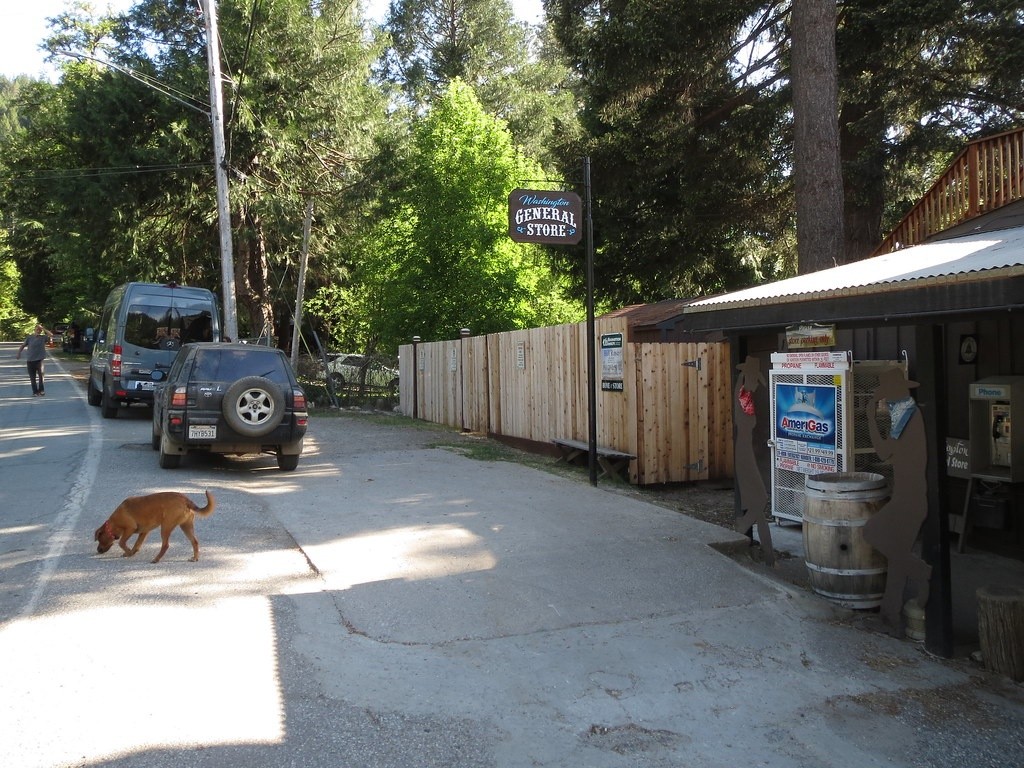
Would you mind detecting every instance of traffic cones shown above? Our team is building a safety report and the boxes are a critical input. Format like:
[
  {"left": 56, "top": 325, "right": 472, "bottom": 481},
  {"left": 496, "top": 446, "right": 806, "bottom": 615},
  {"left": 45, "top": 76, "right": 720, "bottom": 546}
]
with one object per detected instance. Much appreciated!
[{"left": 49, "top": 338, "right": 54, "bottom": 348}]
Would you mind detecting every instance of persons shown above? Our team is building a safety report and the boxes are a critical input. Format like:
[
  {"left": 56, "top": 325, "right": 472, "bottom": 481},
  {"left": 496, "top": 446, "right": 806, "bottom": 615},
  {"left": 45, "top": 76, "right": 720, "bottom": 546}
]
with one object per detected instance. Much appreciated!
[{"left": 16, "top": 323, "right": 53, "bottom": 397}]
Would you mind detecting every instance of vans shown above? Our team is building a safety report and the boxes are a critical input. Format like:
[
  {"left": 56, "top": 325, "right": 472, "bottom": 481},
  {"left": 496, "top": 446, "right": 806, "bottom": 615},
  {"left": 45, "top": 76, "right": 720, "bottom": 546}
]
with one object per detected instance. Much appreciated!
[{"left": 85, "top": 282, "right": 222, "bottom": 418}]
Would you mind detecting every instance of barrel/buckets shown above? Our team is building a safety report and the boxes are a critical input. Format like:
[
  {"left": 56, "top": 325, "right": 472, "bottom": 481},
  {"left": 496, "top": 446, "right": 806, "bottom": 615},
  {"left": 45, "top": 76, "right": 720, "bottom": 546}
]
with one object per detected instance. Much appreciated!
[{"left": 802, "top": 472, "right": 891, "bottom": 609}]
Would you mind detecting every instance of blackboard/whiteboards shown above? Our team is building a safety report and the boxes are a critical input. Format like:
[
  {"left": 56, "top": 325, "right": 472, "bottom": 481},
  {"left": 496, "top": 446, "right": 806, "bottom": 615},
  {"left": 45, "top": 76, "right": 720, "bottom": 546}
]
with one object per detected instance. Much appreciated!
[{"left": 945, "top": 437, "right": 971, "bottom": 480}]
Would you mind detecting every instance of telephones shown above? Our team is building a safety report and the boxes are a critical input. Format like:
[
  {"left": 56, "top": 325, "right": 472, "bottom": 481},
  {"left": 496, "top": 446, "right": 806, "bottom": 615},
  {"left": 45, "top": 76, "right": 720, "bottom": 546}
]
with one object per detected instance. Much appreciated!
[{"left": 990, "top": 404, "right": 1012, "bottom": 467}]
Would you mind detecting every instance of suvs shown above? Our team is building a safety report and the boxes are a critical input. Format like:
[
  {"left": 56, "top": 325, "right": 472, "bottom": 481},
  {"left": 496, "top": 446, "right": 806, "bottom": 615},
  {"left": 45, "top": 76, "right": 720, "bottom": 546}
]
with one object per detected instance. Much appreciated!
[
  {"left": 150, "top": 340, "right": 309, "bottom": 471},
  {"left": 61, "top": 322, "right": 96, "bottom": 355},
  {"left": 49, "top": 323, "right": 69, "bottom": 344},
  {"left": 316, "top": 354, "right": 401, "bottom": 393}
]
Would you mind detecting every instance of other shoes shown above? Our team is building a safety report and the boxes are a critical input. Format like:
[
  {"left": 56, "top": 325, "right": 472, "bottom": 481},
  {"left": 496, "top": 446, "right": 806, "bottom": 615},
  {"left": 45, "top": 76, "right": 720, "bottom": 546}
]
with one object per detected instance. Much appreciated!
[
  {"left": 40, "top": 390, "right": 45, "bottom": 395},
  {"left": 33, "top": 393, "right": 37, "bottom": 397}
]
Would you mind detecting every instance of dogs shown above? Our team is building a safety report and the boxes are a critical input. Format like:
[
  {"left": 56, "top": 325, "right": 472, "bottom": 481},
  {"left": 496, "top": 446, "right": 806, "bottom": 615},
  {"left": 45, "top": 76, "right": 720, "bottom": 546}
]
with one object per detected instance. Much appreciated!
[{"left": 93, "top": 488, "right": 215, "bottom": 564}]
